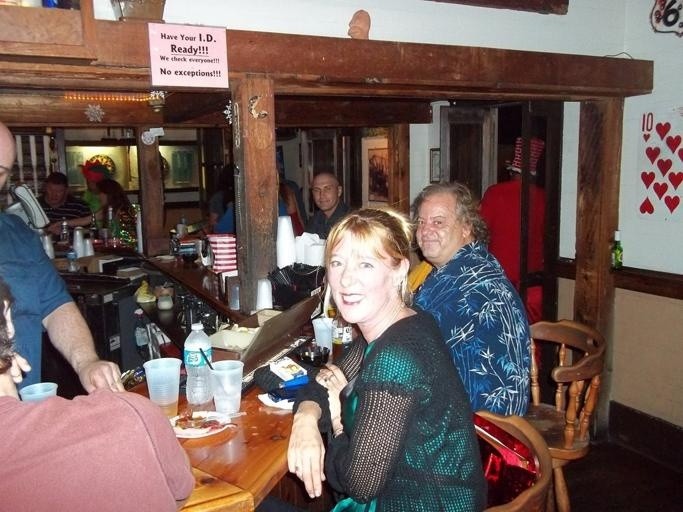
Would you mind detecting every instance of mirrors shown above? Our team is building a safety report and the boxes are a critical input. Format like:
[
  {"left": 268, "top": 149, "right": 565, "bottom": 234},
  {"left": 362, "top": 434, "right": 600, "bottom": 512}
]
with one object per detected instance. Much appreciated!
[{"left": 65, "top": 145, "right": 200, "bottom": 193}]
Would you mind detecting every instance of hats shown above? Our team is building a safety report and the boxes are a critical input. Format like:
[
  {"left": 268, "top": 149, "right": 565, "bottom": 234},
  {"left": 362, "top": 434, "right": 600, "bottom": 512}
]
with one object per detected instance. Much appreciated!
[{"left": 506, "top": 138, "right": 543, "bottom": 176}]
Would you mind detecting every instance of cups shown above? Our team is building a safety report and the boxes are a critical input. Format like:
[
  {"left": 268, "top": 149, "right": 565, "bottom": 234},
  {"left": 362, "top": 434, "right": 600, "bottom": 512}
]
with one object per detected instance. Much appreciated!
[
  {"left": 41, "top": 235, "right": 55, "bottom": 259},
  {"left": 276, "top": 215, "right": 296, "bottom": 269},
  {"left": 136, "top": 211, "right": 143, "bottom": 254},
  {"left": 312, "top": 318, "right": 334, "bottom": 355},
  {"left": 143, "top": 357, "right": 183, "bottom": 420},
  {"left": 73, "top": 227, "right": 84, "bottom": 258},
  {"left": 206, "top": 360, "right": 245, "bottom": 415},
  {"left": 85, "top": 239, "right": 94, "bottom": 257},
  {"left": 19, "top": 382, "right": 58, "bottom": 401},
  {"left": 256, "top": 278, "right": 273, "bottom": 310}
]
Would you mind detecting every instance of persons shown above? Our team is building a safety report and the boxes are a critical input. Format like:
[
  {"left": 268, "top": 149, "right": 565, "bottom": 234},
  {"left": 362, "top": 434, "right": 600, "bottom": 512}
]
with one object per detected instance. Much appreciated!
[
  {"left": 472, "top": 133, "right": 544, "bottom": 372},
  {"left": 302, "top": 171, "right": 354, "bottom": 239},
  {"left": 46, "top": 178, "right": 137, "bottom": 250},
  {"left": 367, "top": 155, "right": 389, "bottom": 201},
  {"left": 287, "top": 207, "right": 489, "bottom": 512},
  {"left": 0, "top": 122, "right": 125, "bottom": 394},
  {"left": 409, "top": 181, "right": 531, "bottom": 419},
  {"left": 0, "top": 272, "right": 196, "bottom": 512},
  {"left": 207, "top": 163, "right": 308, "bottom": 239},
  {"left": 36, "top": 172, "right": 99, "bottom": 244},
  {"left": 78, "top": 154, "right": 122, "bottom": 237}
]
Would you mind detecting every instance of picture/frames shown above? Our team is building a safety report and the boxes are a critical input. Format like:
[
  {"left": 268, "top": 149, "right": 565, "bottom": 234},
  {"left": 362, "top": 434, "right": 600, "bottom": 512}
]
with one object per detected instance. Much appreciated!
[
  {"left": 429, "top": 147, "right": 439, "bottom": 183},
  {"left": 65, "top": 151, "right": 86, "bottom": 187},
  {"left": 367, "top": 147, "right": 388, "bottom": 202},
  {"left": 171, "top": 148, "right": 193, "bottom": 185},
  {"left": 276, "top": 144, "right": 285, "bottom": 180}
]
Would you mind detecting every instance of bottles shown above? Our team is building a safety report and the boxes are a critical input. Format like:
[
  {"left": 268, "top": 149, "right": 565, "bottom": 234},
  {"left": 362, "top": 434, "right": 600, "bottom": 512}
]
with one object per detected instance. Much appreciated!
[
  {"left": 342, "top": 320, "right": 352, "bottom": 348},
  {"left": 87, "top": 211, "right": 99, "bottom": 239},
  {"left": 66, "top": 245, "right": 79, "bottom": 273},
  {"left": 182, "top": 322, "right": 211, "bottom": 405},
  {"left": 41, "top": 231, "right": 55, "bottom": 260},
  {"left": 134, "top": 310, "right": 150, "bottom": 350},
  {"left": 331, "top": 308, "right": 343, "bottom": 343},
  {"left": 83, "top": 232, "right": 95, "bottom": 256},
  {"left": 610, "top": 229, "right": 623, "bottom": 269},
  {"left": 170, "top": 234, "right": 179, "bottom": 255},
  {"left": 108, "top": 207, "right": 118, "bottom": 239},
  {"left": 60, "top": 216, "right": 69, "bottom": 241},
  {"left": 75, "top": 226, "right": 84, "bottom": 258}
]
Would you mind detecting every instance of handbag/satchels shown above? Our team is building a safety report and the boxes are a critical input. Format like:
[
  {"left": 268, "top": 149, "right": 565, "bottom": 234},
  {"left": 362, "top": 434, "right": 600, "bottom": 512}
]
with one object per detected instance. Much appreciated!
[
  {"left": 253, "top": 355, "right": 332, "bottom": 400},
  {"left": 207, "top": 234, "right": 237, "bottom": 274},
  {"left": 473, "top": 410, "right": 542, "bottom": 510}
]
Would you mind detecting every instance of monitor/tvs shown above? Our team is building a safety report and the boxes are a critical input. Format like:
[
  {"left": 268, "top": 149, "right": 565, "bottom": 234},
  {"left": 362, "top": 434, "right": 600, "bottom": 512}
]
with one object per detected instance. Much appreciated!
[{"left": 240, "top": 292, "right": 322, "bottom": 385}]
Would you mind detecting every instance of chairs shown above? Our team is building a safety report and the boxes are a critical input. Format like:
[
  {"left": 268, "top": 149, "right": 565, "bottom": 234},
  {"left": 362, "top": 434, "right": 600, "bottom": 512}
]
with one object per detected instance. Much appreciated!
[
  {"left": 503, "top": 320, "right": 605, "bottom": 512},
  {"left": 475, "top": 410, "right": 553, "bottom": 512}
]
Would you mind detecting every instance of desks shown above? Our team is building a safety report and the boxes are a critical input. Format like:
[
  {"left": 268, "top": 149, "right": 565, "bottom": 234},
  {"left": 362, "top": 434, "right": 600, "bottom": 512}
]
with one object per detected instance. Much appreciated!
[{"left": 125, "top": 308, "right": 362, "bottom": 512}]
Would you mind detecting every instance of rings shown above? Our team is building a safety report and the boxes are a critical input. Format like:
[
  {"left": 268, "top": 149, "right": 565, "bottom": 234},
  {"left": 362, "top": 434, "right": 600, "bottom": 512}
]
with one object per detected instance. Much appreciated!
[
  {"left": 323, "top": 378, "right": 329, "bottom": 384},
  {"left": 329, "top": 374, "right": 334, "bottom": 381}
]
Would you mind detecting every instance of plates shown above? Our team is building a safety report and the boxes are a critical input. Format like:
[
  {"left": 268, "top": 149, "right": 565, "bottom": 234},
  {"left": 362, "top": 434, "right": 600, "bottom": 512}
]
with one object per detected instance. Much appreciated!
[{"left": 169, "top": 411, "right": 232, "bottom": 439}]
